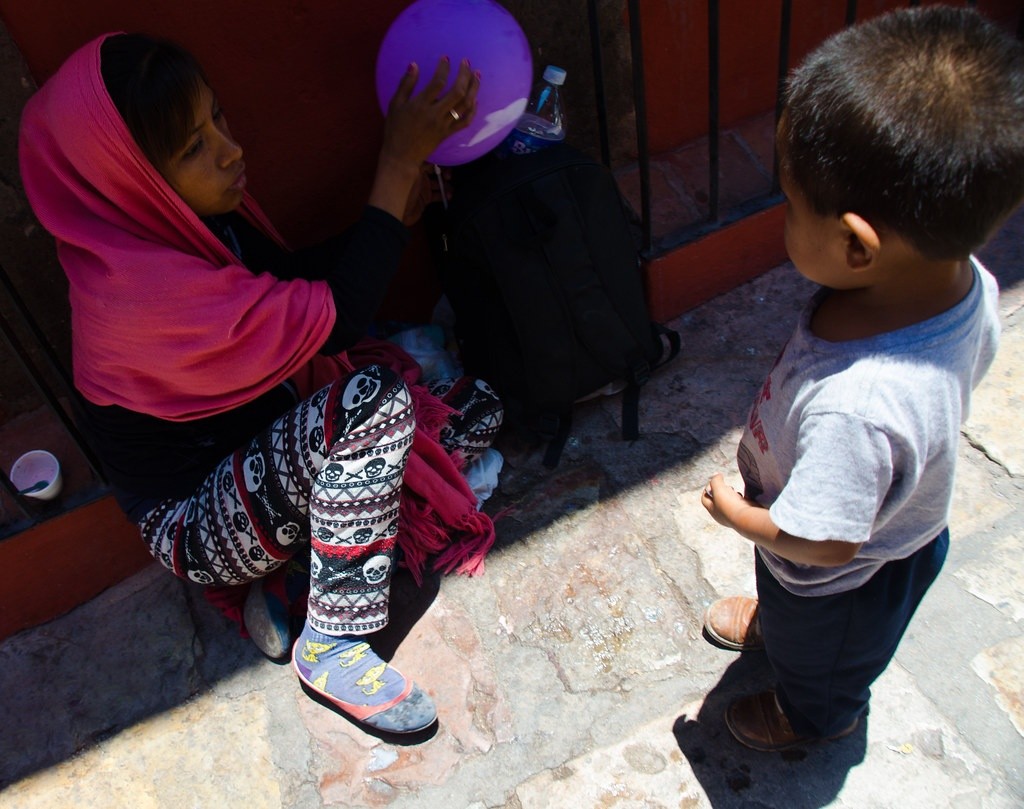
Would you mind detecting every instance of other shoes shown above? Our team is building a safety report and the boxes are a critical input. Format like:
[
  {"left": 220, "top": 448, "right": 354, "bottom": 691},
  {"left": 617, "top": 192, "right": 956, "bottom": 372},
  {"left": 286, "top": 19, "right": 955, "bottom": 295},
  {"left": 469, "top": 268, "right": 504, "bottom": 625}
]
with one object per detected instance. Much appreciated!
[
  {"left": 292, "top": 640, "right": 439, "bottom": 735},
  {"left": 724, "top": 691, "right": 862, "bottom": 752},
  {"left": 705, "top": 595, "right": 782, "bottom": 653},
  {"left": 243, "top": 580, "right": 294, "bottom": 662}
]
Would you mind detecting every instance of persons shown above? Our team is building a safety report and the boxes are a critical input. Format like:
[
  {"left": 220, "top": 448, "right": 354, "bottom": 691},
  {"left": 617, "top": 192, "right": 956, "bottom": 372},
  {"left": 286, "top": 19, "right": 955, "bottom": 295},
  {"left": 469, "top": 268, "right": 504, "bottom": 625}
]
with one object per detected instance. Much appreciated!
[
  {"left": 700, "top": 7, "right": 1023, "bottom": 751},
  {"left": 20, "top": 31, "right": 502, "bottom": 735}
]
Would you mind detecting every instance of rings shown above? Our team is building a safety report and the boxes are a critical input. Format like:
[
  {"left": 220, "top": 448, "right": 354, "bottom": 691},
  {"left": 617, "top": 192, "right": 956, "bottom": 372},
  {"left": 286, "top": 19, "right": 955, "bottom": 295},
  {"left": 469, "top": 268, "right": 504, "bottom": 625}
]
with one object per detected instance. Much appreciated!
[{"left": 449, "top": 107, "right": 461, "bottom": 123}]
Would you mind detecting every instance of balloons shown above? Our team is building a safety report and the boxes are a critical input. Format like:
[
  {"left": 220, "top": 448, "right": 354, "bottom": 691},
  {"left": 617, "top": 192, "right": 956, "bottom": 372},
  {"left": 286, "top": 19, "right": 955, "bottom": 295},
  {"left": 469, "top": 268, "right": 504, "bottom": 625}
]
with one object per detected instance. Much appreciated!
[{"left": 375, "top": 1, "right": 534, "bottom": 167}]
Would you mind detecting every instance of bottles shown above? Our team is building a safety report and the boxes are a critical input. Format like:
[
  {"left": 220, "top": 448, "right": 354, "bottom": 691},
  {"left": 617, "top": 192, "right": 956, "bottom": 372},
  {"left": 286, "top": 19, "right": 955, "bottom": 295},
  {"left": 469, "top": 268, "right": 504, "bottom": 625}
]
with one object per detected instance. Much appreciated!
[{"left": 505, "top": 63, "right": 571, "bottom": 155}]
